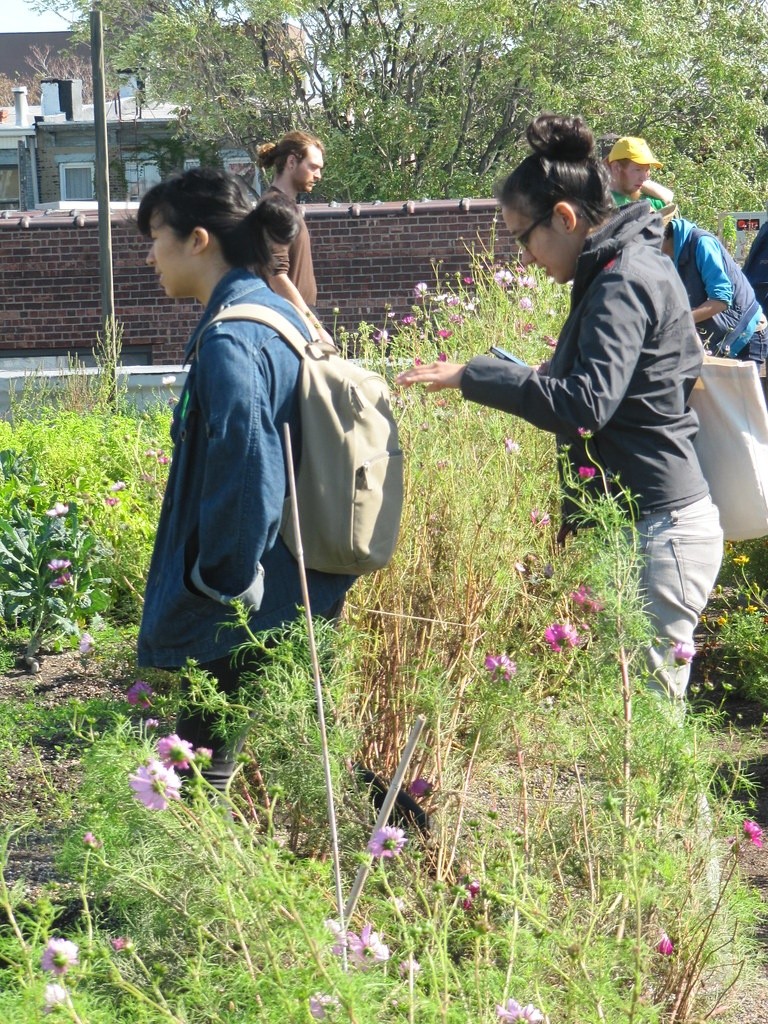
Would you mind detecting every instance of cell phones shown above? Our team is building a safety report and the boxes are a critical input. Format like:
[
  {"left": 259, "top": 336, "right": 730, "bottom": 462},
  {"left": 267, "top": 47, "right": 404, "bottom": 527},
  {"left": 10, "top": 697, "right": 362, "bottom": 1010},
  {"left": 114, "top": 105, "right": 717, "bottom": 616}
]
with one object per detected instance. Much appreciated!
[{"left": 489, "top": 346, "right": 528, "bottom": 368}]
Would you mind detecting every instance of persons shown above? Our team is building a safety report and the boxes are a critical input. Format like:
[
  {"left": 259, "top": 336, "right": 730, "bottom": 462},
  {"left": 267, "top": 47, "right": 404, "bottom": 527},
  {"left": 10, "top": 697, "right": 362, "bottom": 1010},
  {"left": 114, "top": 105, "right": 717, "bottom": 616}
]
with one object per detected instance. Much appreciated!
[
  {"left": 136, "top": 165, "right": 429, "bottom": 838},
  {"left": 254, "top": 130, "right": 336, "bottom": 349},
  {"left": 390, "top": 114, "right": 723, "bottom": 905},
  {"left": 604, "top": 136, "right": 768, "bottom": 409}
]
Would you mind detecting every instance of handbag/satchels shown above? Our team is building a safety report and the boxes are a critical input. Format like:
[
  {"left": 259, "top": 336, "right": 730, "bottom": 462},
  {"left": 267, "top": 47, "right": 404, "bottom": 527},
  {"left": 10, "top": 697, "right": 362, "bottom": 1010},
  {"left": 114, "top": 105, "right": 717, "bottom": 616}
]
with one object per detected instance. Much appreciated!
[{"left": 686, "top": 355, "right": 768, "bottom": 541}]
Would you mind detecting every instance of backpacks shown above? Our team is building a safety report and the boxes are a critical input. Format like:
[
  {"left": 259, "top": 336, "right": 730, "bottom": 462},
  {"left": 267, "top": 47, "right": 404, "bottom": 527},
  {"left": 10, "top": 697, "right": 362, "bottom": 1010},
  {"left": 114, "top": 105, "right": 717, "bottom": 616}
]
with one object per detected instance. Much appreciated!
[{"left": 196, "top": 302, "right": 403, "bottom": 575}]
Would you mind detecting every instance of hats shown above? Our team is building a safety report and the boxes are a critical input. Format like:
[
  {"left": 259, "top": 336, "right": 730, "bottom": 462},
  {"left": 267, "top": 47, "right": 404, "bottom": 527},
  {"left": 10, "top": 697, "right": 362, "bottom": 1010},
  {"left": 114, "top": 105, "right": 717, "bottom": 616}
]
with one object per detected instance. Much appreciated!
[
  {"left": 608, "top": 137, "right": 662, "bottom": 168},
  {"left": 649, "top": 203, "right": 677, "bottom": 227}
]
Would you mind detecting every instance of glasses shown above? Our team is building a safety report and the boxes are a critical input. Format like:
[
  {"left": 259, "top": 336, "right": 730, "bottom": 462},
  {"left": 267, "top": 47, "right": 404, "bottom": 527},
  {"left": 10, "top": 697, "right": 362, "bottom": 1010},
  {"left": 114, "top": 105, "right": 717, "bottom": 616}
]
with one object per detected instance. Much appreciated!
[{"left": 515, "top": 206, "right": 583, "bottom": 251}]
